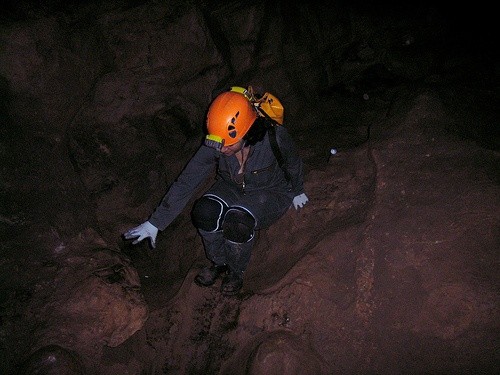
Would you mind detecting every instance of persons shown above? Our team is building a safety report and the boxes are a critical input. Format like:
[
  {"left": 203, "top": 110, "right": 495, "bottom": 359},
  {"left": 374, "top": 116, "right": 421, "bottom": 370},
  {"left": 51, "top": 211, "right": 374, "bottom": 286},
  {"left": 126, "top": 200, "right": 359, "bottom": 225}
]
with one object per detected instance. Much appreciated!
[{"left": 122, "top": 84, "right": 310, "bottom": 297}]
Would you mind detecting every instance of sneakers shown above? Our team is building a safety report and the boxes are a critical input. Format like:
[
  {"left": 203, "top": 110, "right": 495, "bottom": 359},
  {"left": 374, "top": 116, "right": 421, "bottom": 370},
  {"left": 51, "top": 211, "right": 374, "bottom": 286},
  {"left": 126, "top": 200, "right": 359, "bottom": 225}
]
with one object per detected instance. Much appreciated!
[{"left": 194, "top": 262, "right": 228, "bottom": 286}]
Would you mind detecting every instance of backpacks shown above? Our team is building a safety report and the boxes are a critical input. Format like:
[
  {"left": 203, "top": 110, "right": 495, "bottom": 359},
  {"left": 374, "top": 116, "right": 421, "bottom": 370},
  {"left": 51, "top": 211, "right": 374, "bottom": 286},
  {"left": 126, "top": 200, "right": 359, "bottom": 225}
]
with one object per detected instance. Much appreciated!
[{"left": 246, "top": 84, "right": 284, "bottom": 129}]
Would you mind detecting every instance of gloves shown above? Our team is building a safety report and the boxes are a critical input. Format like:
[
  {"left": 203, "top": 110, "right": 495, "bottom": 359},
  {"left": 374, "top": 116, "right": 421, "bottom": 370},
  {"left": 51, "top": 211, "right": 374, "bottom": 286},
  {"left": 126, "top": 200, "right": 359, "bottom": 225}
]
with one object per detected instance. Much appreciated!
[
  {"left": 293, "top": 192, "right": 309, "bottom": 211},
  {"left": 123, "top": 221, "right": 159, "bottom": 250}
]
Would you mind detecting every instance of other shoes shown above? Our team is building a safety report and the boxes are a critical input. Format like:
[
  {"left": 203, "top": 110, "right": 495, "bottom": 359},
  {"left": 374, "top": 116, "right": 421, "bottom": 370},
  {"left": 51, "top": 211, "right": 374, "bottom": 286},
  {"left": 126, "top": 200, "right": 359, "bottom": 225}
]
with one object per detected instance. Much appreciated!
[{"left": 220, "top": 269, "right": 244, "bottom": 294}]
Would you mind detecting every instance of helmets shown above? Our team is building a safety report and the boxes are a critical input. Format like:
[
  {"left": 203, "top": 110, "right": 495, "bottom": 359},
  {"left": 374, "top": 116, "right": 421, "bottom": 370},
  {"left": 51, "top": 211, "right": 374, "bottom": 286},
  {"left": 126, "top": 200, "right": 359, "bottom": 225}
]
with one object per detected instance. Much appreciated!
[{"left": 205, "top": 91, "right": 257, "bottom": 151}]
